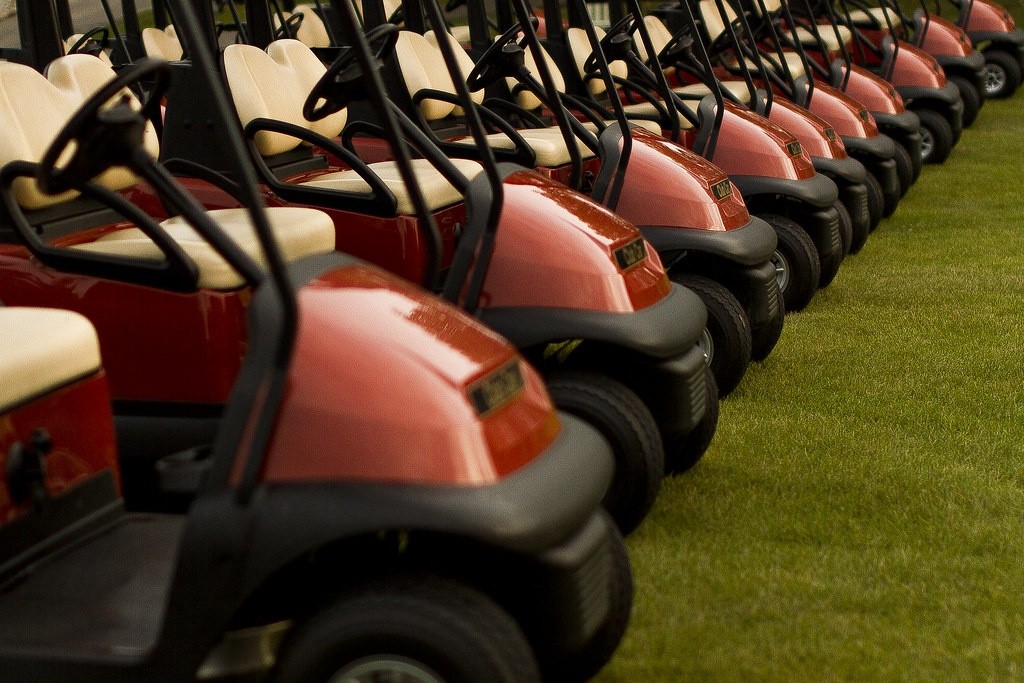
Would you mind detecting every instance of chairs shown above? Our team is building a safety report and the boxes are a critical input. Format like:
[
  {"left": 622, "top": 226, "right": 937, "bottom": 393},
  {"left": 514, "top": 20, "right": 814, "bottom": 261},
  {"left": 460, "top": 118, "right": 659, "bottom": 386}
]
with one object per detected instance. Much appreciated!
[{"left": 0, "top": 0, "right": 900, "bottom": 546}]
